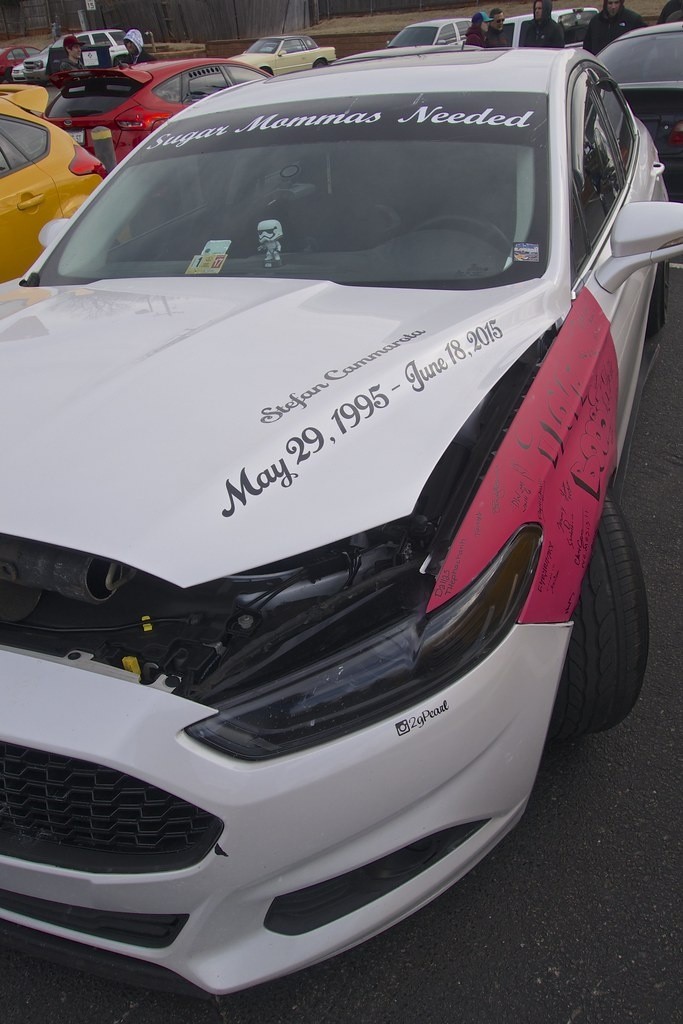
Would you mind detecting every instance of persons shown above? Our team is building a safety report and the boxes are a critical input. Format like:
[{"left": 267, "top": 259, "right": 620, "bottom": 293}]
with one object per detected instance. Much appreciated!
[
  {"left": 59, "top": 35, "right": 90, "bottom": 77},
  {"left": 583, "top": 0, "right": 649, "bottom": 56},
  {"left": 656, "top": 0, "right": 683, "bottom": 25},
  {"left": 257, "top": 219, "right": 284, "bottom": 268},
  {"left": 465, "top": 7, "right": 509, "bottom": 48},
  {"left": 523, "top": 0, "right": 565, "bottom": 48},
  {"left": 117, "top": 30, "right": 158, "bottom": 67}
]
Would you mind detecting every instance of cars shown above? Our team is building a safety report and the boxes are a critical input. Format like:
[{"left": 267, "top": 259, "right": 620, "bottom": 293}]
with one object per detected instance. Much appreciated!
[
  {"left": 11, "top": 62, "right": 27, "bottom": 84},
  {"left": 42, "top": 57, "right": 273, "bottom": 173},
  {"left": 227, "top": 34, "right": 336, "bottom": 77},
  {"left": 594, "top": 22, "right": 683, "bottom": 204},
  {"left": 0, "top": 44, "right": 41, "bottom": 84},
  {"left": 0, "top": 83, "right": 110, "bottom": 285},
  {"left": 0, "top": 52, "right": 683, "bottom": 1003},
  {"left": 385, "top": 18, "right": 472, "bottom": 48}
]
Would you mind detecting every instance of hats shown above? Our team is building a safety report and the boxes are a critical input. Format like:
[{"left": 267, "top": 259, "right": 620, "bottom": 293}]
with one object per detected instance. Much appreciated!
[
  {"left": 64, "top": 36, "right": 86, "bottom": 48},
  {"left": 472, "top": 12, "right": 494, "bottom": 23}
]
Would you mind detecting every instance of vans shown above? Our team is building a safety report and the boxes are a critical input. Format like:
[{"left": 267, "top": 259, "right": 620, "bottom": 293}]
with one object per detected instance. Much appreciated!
[
  {"left": 22, "top": 29, "right": 133, "bottom": 86},
  {"left": 502, "top": 7, "right": 599, "bottom": 49}
]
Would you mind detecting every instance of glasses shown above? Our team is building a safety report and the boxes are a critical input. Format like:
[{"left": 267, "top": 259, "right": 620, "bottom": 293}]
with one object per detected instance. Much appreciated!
[{"left": 496, "top": 18, "right": 504, "bottom": 23}]
